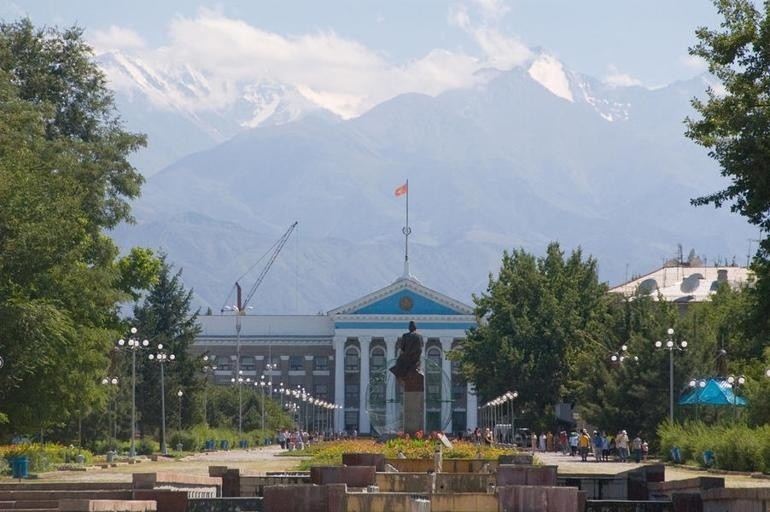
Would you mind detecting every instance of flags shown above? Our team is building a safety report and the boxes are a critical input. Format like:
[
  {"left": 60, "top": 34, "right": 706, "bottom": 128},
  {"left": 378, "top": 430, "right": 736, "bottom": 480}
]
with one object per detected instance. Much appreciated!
[{"left": 394, "top": 184, "right": 407, "bottom": 197}]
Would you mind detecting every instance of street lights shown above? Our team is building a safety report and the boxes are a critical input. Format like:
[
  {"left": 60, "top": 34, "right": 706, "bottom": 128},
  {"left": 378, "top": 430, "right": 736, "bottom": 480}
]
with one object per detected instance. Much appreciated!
[
  {"left": 118, "top": 325, "right": 150, "bottom": 459},
  {"left": 687, "top": 377, "right": 708, "bottom": 423},
  {"left": 726, "top": 373, "right": 748, "bottom": 426},
  {"left": 176, "top": 388, "right": 183, "bottom": 447},
  {"left": 265, "top": 360, "right": 277, "bottom": 384},
  {"left": 253, "top": 373, "right": 272, "bottom": 436},
  {"left": 197, "top": 349, "right": 218, "bottom": 426},
  {"left": 273, "top": 381, "right": 346, "bottom": 445},
  {"left": 653, "top": 327, "right": 689, "bottom": 425},
  {"left": 148, "top": 340, "right": 176, "bottom": 453},
  {"left": 609, "top": 344, "right": 645, "bottom": 373},
  {"left": 222, "top": 304, "right": 253, "bottom": 377},
  {"left": 101, "top": 375, "right": 121, "bottom": 451},
  {"left": 231, "top": 366, "right": 249, "bottom": 437},
  {"left": 477, "top": 391, "right": 521, "bottom": 449}
]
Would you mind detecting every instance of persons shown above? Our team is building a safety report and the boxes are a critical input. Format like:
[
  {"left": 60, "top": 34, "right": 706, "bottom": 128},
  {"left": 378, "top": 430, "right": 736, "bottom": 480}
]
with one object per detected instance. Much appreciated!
[
  {"left": 458, "top": 431, "right": 464, "bottom": 441},
  {"left": 483, "top": 427, "right": 494, "bottom": 446},
  {"left": 467, "top": 429, "right": 472, "bottom": 440},
  {"left": 280, "top": 427, "right": 334, "bottom": 449},
  {"left": 530, "top": 428, "right": 648, "bottom": 463},
  {"left": 473, "top": 426, "right": 482, "bottom": 442},
  {"left": 397, "top": 321, "right": 424, "bottom": 377},
  {"left": 352, "top": 429, "right": 358, "bottom": 440}
]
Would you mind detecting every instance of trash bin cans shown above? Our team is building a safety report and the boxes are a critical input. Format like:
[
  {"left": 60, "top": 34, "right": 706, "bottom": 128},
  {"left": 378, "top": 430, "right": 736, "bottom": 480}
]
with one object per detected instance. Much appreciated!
[
  {"left": 205, "top": 440, "right": 249, "bottom": 449},
  {"left": 12, "top": 458, "right": 29, "bottom": 478},
  {"left": 705, "top": 451, "right": 713, "bottom": 465},
  {"left": 671, "top": 447, "right": 681, "bottom": 463}
]
